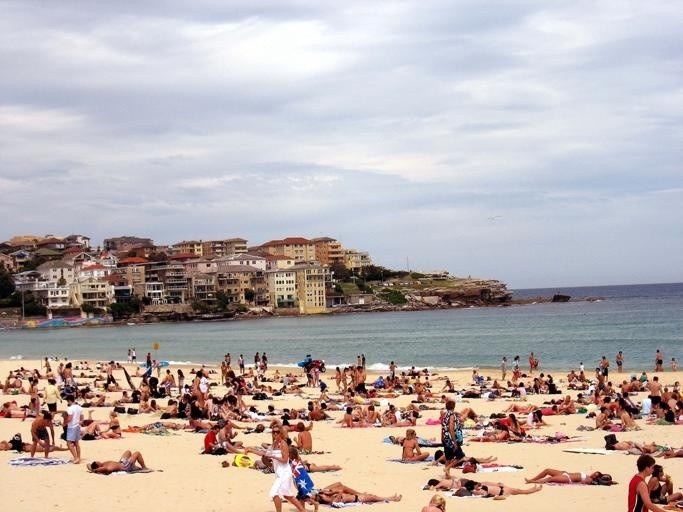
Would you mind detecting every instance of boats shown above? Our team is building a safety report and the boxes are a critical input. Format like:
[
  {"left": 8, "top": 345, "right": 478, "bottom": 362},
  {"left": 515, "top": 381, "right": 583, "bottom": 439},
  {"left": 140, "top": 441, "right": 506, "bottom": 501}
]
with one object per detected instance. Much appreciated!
[
  {"left": 497, "top": 303, "right": 511, "bottom": 307},
  {"left": 591, "top": 298, "right": 602, "bottom": 302},
  {"left": 332, "top": 303, "right": 339, "bottom": 308}
]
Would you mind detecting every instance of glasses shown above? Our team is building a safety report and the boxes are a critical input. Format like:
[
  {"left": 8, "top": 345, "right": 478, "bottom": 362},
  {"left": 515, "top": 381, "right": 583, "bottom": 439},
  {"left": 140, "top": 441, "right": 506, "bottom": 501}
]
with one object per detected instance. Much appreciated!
[{"left": 272, "top": 430, "right": 280, "bottom": 434}]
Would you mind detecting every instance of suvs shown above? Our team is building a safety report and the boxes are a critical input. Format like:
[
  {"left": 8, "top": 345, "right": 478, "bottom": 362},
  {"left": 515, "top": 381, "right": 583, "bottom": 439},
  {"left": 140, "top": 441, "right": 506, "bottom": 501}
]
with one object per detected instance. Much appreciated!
[{"left": 302, "top": 360, "right": 325, "bottom": 374}]
